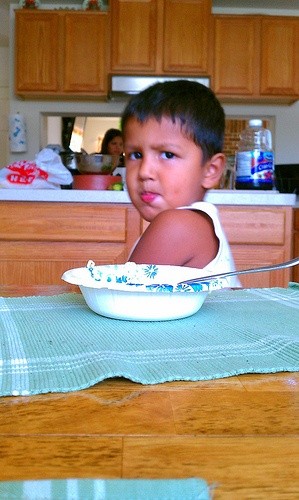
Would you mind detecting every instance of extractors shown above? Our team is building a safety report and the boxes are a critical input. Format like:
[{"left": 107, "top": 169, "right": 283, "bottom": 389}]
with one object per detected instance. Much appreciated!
[{"left": 108, "top": 75, "right": 210, "bottom": 99}]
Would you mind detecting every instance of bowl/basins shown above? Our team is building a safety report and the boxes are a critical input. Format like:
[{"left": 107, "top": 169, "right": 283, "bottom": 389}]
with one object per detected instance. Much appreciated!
[
  {"left": 62, "top": 263, "right": 229, "bottom": 322},
  {"left": 57, "top": 151, "right": 122, "bottom": 190}
]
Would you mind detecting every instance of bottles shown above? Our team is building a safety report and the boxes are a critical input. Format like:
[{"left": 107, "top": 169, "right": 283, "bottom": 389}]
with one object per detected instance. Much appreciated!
[{"left": 233, "top": 119, "right": 274, "bottom": 191}]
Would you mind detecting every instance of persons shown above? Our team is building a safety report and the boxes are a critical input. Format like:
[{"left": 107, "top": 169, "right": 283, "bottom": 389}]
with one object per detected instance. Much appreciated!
[
  {"left": 121, "top": 80, "right": 241, "bottom": 288},
  {"left": 100, "top": 129, "right": 122, "bottom": 155}
]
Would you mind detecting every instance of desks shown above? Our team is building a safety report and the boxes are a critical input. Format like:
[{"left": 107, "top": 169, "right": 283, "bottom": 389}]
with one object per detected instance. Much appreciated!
[{"left": 0, "top": 281, "right": 299, "bottom": 500}]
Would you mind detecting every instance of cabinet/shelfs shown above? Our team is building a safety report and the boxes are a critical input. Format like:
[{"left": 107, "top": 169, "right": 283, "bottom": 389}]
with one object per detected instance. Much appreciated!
[
  {"left": 15, "top": 9, "right": 110, "bottom": 97},
  {"left": 109, "top": 0, "right": 214, "bottom": 76},
  {"left": 210, "top": 15, "right": 299, "bottom": 103}
]
